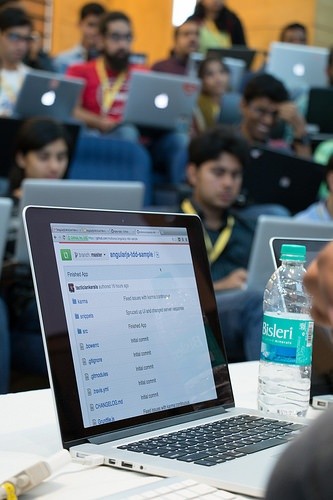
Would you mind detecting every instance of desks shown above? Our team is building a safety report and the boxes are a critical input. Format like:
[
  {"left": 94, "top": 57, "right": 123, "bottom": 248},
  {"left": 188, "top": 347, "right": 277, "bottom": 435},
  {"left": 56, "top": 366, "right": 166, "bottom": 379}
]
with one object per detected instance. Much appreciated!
[{"left": 0, "top": 360, "right": 333, "bottom": 500}]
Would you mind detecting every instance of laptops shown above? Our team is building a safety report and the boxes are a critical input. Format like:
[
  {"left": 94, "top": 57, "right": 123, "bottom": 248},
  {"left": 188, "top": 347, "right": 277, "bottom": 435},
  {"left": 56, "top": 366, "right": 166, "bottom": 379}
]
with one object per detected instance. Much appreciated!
[
  {"left": 7, "top": 72, "right": 84, "bottom": 122},
  {"left": 0, "top": 180, "right": 333, "bottom": 498},
  {"left": 120, "top": 42, "right": 333, "bottom": 133},
  {"left": 233, "top": 147, "right": 328, "bottom": 219}
]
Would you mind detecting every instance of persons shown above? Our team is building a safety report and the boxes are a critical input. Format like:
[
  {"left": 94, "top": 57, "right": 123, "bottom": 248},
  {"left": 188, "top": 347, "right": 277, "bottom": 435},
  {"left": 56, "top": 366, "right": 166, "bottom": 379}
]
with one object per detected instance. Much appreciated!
[
  {"left": 283, "top": 24, "right": 307, "bottom": 45},
  {"left": 150, "top": 127, "right": 264, "bottom": 362},
  {"left": 295, "top": 155, "right": 333, "bottom": 221},
  {"left": 3, "top": 115, "right": 69, "bottom": 206},
  {"left": 66, "top": 12, "right": 190, "bottom": 182},
  {"left": 187, "top": 0, "right": 246, "bottom": 55},
  {"left": 236, "top": 74, "right": 312, "bottom": 159},
  {"left": 0, "top": 8, "right": 35, "bottom": 115},
  {"left": 192, "top": 58, "right": 230, "bottom": 127},
  {"left": 55, "top": 3, "right": 106, "bottom": 75},
  {"left": 151, "top": 22, "right": 199, "bottom": 75}
]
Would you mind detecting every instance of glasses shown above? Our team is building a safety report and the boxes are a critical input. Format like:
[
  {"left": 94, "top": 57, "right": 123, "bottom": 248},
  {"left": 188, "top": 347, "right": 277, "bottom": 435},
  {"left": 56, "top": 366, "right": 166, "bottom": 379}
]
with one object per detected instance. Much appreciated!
[
  {"left": 7, "top": 33, "right": 33, "bottom": 44},
  {"left": 111, "top": 34, "right": 133, "bottom": 42}
]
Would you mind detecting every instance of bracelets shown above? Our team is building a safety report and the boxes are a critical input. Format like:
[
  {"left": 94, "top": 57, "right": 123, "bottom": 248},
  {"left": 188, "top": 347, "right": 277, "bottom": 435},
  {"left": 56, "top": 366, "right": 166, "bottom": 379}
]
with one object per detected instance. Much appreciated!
[{"left": 295, "top": 135, "right": 309, "bottom": 143}]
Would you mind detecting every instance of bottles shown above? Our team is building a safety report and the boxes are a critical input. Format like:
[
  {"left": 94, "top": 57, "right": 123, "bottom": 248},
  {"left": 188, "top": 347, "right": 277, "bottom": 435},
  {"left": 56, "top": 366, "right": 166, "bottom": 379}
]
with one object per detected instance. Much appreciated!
[{"left": 256, "top": 244, "right": 314, "bottom": 418}]
